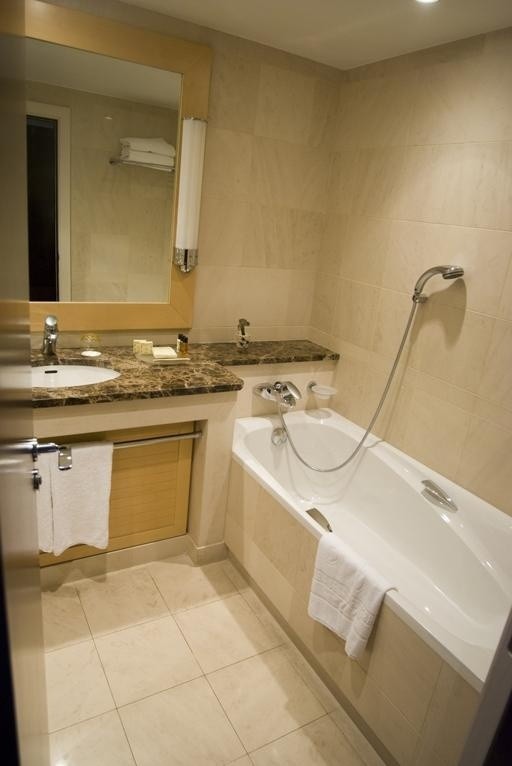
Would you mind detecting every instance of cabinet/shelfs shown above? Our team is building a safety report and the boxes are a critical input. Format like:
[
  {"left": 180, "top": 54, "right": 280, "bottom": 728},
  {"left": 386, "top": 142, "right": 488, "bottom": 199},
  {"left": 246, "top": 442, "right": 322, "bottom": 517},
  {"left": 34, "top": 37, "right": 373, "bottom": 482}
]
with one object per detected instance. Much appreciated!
[{"left": 41, "top": 421, "right": 199, "bottom": 565}]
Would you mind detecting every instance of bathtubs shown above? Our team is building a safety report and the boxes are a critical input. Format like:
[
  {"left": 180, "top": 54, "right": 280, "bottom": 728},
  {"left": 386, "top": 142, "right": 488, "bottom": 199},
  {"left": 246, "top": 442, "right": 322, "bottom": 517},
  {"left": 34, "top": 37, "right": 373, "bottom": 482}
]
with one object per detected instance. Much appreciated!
[{"left": 231, "top": 405, "right": 512, "bottom": 694}]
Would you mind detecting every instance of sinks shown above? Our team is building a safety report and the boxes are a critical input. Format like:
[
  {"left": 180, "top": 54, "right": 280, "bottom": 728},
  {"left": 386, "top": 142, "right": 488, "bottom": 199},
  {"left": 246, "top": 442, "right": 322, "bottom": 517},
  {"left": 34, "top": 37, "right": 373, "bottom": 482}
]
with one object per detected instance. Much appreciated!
[{"left": 32, "top": 358, "right": 122, "bottom": 387}]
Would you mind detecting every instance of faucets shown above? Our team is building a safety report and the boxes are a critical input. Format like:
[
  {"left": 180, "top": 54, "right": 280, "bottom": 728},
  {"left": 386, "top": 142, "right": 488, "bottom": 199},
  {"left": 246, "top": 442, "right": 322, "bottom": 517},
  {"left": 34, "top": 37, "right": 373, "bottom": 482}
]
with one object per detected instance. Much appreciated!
[{"left": 42, "top": 315, "right": 59, "bottom": 355}]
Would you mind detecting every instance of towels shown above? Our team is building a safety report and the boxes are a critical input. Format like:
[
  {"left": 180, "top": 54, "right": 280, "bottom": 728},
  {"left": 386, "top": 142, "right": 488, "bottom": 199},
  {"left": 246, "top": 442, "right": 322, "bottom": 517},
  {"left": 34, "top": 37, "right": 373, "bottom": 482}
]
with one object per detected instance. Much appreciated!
[
  {"left": 118, "top": 131, "right": 177, "bottom": 156},
  {"left": 119, "top": 148, "right": 174, "bottom": 172},
  {"left": 52, "top": 440, "right": 116, "bottom": 559},
  {"left": 306, "top": 533, "right": 403, "bottom": 661},
  {"left": 38, "top": 446, "right": 59, "bottom": 555}
]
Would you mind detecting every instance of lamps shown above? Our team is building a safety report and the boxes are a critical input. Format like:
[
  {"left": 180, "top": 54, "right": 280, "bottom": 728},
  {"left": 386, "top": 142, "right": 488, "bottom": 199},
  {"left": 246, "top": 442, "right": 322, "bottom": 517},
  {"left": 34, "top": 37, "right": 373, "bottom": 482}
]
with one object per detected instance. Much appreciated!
[{"left": 172, "top": 115, "right": 210, "bottom": 273}]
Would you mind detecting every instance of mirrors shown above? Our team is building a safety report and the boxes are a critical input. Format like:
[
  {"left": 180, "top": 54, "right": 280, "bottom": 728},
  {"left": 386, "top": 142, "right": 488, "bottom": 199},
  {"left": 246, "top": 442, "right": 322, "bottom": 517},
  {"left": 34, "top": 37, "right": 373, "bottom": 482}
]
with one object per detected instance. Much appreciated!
[{"left": 1, "top": 29, "right": 187, "bottom": 306}]
